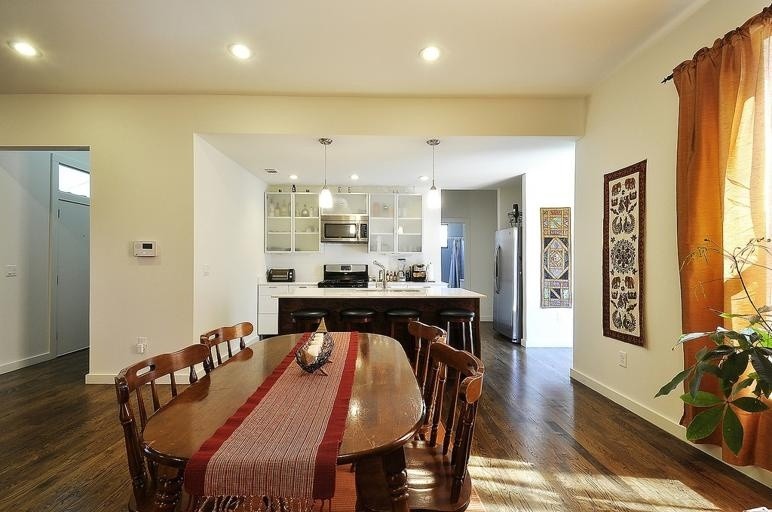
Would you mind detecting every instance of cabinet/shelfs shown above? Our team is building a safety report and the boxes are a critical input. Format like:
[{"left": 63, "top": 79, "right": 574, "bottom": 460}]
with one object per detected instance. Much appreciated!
[
  {"left": 263, "top": 184, "right": 322, "bottom": 254},
  {"left": 368, "top": 189, "right": 425, "bottom": 253},
  {"left": 259, "top": 285, "right": 288, "bottom": 334}
]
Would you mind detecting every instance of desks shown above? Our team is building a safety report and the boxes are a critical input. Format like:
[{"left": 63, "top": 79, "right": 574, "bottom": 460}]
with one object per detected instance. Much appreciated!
[{"left": 141, "top": 332, "right": 427, "bottom": 512}]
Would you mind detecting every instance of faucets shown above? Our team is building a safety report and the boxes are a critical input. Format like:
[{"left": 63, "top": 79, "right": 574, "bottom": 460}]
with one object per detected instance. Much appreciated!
[{"left": 372, "top": 260, "right": 389, "bottom": 291}]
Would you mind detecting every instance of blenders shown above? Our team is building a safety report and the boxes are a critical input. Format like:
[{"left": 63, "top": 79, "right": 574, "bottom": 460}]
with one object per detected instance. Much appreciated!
[{"left": 396, "top": 259, "right": 406, "bottom": 281}]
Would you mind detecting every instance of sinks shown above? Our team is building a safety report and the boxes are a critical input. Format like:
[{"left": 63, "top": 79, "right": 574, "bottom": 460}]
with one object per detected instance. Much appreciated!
[{"left": 356, "top": 289, "right": 422, "bottom": 293}]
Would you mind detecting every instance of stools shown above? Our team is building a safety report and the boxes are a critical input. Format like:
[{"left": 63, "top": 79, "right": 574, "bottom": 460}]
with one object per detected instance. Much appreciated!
[
  {"left": 386, "top": 309, "right": 421, "bottom": 344},
  {"left": 442, "top": 310, "right": 478, "bottom": 388},
  {"left": 289, "top": 307, "right": 329, "bottom": 333},
  {"left": 339, "top": 307, "right": 376, "bottom": 331}
]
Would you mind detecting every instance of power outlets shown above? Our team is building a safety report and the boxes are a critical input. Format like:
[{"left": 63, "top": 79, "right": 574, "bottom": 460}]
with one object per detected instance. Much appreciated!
[{"left": 618, "top": 352, "right": 627, "bottom": 367}]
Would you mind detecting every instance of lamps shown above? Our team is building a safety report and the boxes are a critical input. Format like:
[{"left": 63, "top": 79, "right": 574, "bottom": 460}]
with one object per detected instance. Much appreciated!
[
  {"left": 426, "top": 139, "right": 441, "bottom": 209},
  {"left": 441, "top": 223, "right": 449, "bottom": 248},
  {"left": 318, "top": 138, "right": 334, "bottom": 210}
]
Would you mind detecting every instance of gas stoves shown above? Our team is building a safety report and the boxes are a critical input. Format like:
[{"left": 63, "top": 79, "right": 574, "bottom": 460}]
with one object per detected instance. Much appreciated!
[{"left": 320, "top": 279, "right": 367, "bottom": 288}]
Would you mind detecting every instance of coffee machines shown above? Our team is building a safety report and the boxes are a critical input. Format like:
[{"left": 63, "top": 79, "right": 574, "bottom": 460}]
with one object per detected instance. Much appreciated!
[{"left": 411, "top": 263, "right": 427, "bottom": 281}]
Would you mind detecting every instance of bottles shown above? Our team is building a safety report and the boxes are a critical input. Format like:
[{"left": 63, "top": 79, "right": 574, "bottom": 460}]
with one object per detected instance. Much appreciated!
[
  {"left": 292, "top": 184, "right": 296, "bottom": 192},
  {"left": 385, "top": 270, "right": 396, "bottom": 282},
  {"left": 268, "top": 199, "right": 316, "bottom": 217}
]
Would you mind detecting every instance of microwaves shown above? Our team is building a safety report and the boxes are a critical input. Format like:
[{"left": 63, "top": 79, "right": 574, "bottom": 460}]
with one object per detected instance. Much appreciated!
[{"left": 320, "top": 213, "right": 369, "bottom": 243}]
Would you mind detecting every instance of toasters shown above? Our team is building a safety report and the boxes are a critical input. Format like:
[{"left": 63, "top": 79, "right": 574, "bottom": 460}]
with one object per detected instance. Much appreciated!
[{"left": 267, "top": 267, "right": 295, "bottom": 282}]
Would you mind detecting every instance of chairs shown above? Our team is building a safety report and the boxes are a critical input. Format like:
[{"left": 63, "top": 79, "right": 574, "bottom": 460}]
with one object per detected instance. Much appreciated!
[
  {"left": 348, "top": 339, "right": 485, "bottom": 512},
  {"left": 407, "top": 314, "right": 448, "bottom": 418},
  {"left": 200, "top": 323, "right": 254, "bottom": 369},
  {"left": 116, "top": 344, "right": 212, "bottom": 512}
]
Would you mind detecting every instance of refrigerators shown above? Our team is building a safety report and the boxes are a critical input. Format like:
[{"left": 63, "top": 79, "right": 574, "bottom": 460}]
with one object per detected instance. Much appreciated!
[{"left": 492, "top": 228, "right": 519, "bottom": 342}]
